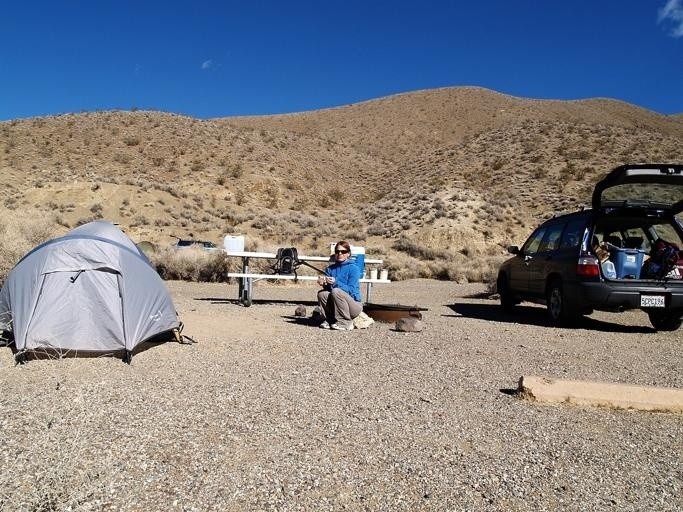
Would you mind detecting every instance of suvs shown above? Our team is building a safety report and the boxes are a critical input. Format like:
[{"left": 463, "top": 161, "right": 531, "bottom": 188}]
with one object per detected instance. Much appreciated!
[
  {"left": 169, "top": 234, "right": 226, "bottom": 255},
  {"left": 495, "top": 162, "right": 683, "bottom": 336}
]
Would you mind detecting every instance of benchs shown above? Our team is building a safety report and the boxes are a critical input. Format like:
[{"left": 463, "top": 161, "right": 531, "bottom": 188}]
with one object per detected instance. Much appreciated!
[{"left": 226, "top": 273, "right": 390, "bottom": 307}]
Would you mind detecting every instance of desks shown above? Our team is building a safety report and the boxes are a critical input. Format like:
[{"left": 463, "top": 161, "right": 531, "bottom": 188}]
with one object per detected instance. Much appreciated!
[{"left": 222, "top": 252, "right": 382, "bottom": 307}]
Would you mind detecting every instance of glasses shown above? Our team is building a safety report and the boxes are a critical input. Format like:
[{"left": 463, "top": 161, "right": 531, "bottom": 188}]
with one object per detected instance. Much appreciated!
[{"left": 335, "top": 250, "right": 349, "bottom": 254}]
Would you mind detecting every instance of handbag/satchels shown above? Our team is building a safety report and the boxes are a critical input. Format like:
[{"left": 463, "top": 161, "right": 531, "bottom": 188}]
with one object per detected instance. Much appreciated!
[{"left": 278, "top": 247, "right": 298, "bottom": 275}]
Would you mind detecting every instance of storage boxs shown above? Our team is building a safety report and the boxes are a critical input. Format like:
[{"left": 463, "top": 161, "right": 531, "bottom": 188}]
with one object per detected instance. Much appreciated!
[{"left": 610, "top": 250, "right": 644, "bottom": 280}]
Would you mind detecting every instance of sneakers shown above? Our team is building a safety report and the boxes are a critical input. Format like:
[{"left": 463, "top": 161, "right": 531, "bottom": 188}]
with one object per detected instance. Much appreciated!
[
  {"left": 331, "top": 319, "right": 354, "bottom": 330},
  {"left": 320, "top": 319, "right": 337, "bottom": 329}
]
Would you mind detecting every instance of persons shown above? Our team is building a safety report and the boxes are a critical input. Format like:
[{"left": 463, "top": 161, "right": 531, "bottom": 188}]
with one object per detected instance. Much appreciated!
[{"left": 315, "top": 240, "right": 363, "bottom": 330}]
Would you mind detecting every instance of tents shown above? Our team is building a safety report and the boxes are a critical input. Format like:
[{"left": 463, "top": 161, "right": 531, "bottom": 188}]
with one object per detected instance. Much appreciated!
[{"left": 0, "top": 220, "right": 185, "bottom": 362}]
